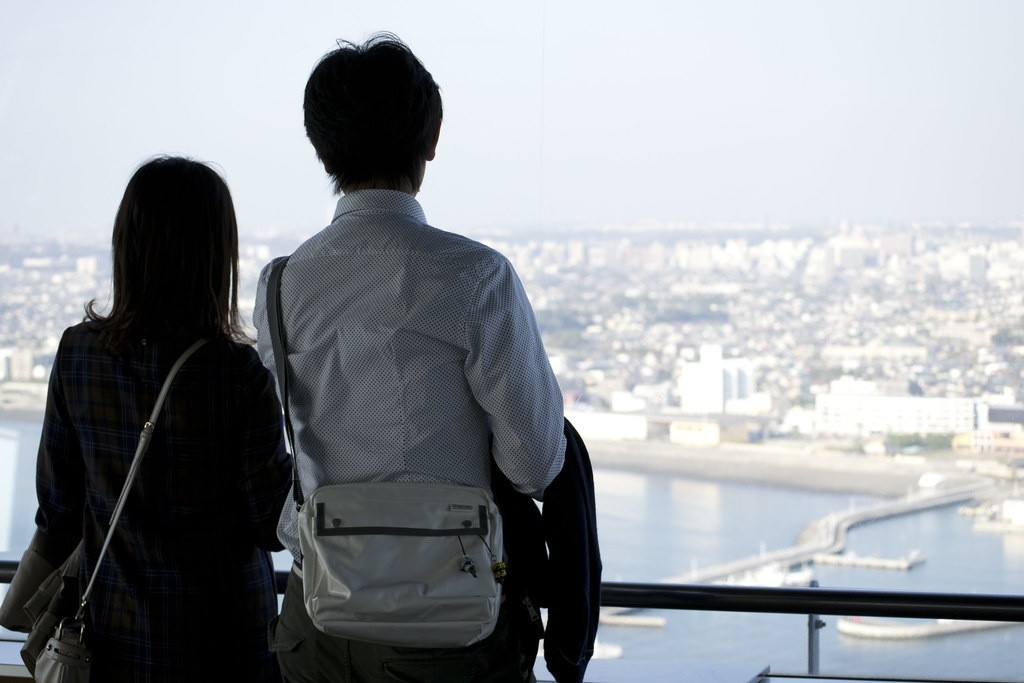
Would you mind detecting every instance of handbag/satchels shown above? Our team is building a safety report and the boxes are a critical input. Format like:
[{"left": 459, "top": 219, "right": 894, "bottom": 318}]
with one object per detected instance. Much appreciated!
[
  {"left": 35, "top": 618, "right": 91, "bottom": 683},
  {"left": 297, "top": 480, "right": 509, "bottom": 650}
]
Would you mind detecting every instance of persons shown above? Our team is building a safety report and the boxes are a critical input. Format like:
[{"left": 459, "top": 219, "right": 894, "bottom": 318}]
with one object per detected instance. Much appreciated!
[
  {"left": 2, "top": 155, "right": 296, "bottom": 683},
  {"left": 254, "top": 31, "right": 604, "bottom": 683}
]
